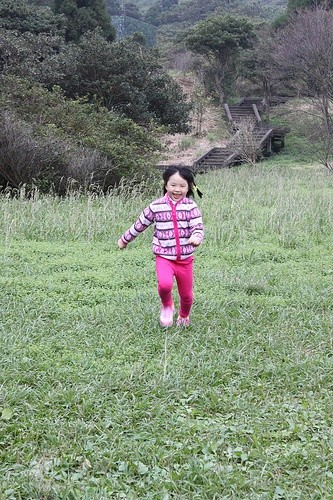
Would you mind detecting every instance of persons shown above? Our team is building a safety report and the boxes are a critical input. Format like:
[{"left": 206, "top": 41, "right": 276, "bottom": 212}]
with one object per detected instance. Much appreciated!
[{"left": 118, "top": 165, "right": 204, "bottom": 328}]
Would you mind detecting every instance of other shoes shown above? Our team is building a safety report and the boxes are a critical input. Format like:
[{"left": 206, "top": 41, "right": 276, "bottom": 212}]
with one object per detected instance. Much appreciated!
[
  {"left": 161, "top": 304, "right": 174, "bottom": 327},
  {"left": 177, "top": 315, "right": 189, "bottom": 327}
]
